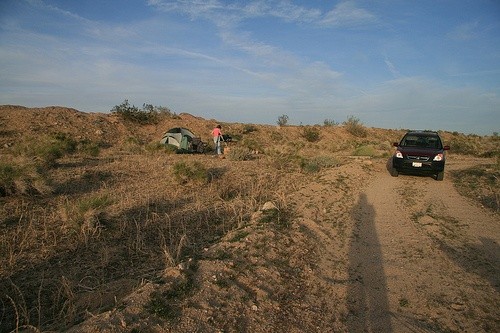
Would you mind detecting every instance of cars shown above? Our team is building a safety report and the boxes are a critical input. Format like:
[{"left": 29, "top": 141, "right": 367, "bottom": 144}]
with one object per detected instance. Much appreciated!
[{"left": 390, "top": 130, "right": 446, "bottom": 180}]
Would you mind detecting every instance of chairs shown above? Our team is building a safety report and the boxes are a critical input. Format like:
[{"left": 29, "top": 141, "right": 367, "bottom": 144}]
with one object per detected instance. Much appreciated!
[
  {"left": 191, "top": 138, "right": 207, "bottom": 155},
  {"left": 404, "top": 139, "right": 416, "bottom": 146},
  {"left": 425, "top": 141, "right": 438, "bottom": 147}
]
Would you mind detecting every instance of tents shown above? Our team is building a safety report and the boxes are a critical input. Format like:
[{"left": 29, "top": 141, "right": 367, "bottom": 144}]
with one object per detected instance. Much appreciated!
[{"left": 160, "top": 127, "right": 202, "bottom": 150}]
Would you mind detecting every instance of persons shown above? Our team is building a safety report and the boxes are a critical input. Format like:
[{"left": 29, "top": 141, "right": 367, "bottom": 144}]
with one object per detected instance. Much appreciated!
[{"left": 210, "top": 125, "right": 223, "bottom": 155}]
